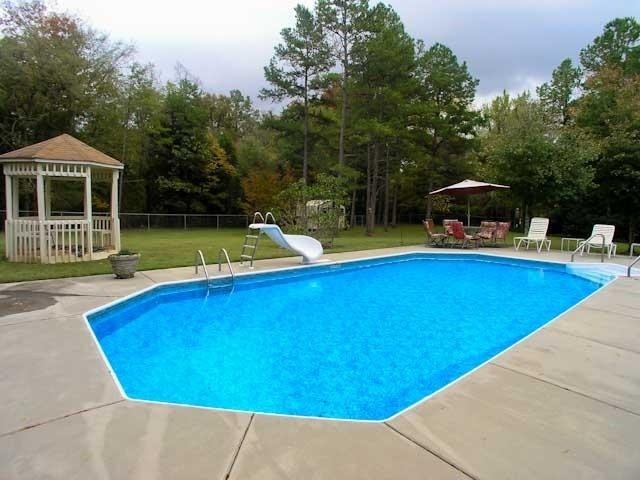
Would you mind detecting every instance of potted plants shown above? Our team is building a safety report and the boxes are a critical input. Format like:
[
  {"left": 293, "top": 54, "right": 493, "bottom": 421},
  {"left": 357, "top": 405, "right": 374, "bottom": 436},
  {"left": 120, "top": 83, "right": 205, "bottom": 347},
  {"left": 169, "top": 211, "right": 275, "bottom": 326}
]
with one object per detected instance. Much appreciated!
[{"left": 107, "top": 248, "right": 140, "bottom": 279}]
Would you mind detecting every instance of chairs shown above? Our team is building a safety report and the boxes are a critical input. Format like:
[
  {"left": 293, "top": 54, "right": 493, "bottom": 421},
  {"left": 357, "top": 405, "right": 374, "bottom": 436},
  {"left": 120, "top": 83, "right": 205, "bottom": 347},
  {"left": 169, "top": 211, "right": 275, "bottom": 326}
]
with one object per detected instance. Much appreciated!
[
  {"left": 423, "top": 218, "right": 511, "bottom": 248},
  {"left": 513, "top": 217, "right": 552, "bottom": 252},
  {"left": 579, "top": 223, "right": 616, "bottom": 259}
]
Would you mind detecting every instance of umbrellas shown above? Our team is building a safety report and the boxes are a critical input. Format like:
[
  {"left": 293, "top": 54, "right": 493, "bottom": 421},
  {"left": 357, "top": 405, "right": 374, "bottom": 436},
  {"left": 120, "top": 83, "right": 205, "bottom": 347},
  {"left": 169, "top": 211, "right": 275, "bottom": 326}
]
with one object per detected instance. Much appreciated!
[{"left": 429, "top": 179, "right": 510, "bottom": 234}]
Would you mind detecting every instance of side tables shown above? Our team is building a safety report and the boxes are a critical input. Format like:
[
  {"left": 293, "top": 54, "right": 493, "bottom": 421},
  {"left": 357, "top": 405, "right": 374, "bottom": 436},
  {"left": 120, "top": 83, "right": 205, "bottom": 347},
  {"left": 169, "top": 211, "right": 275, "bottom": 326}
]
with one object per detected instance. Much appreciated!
[{"left": 561, "top": 237, "right": 585, "bottom": 252}]
